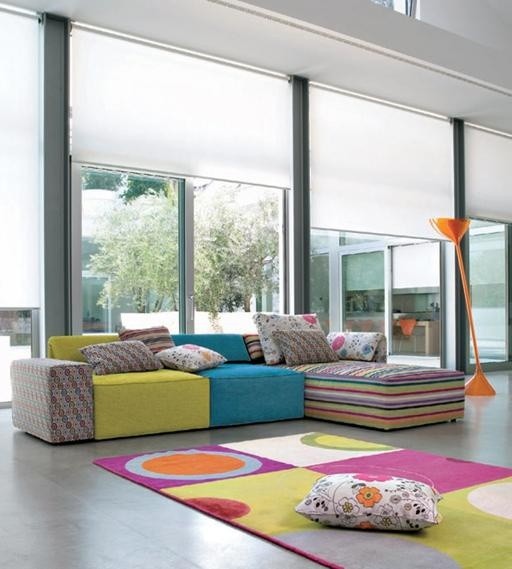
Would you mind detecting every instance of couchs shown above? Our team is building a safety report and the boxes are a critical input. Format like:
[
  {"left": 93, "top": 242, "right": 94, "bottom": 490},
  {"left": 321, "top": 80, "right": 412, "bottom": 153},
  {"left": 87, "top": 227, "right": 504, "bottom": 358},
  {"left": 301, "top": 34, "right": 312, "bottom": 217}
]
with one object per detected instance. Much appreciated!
[{"left": 10, "top": 331, "right": 466, "bottom": 444}]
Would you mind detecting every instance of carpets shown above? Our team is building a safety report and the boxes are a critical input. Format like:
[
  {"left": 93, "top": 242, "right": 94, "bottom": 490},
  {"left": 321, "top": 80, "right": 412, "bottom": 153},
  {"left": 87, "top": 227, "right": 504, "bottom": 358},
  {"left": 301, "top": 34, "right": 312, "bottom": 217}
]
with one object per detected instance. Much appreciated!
[{"left": 90, "top": 431, "right": 512, "bottom": 569}]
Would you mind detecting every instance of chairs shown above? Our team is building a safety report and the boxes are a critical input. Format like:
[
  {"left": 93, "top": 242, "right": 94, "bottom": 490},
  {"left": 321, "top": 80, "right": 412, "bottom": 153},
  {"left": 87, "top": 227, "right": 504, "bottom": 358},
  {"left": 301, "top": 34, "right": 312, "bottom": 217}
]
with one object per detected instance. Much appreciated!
[{"left": 346, "top": 319, "right": 417, "bottom": 354}]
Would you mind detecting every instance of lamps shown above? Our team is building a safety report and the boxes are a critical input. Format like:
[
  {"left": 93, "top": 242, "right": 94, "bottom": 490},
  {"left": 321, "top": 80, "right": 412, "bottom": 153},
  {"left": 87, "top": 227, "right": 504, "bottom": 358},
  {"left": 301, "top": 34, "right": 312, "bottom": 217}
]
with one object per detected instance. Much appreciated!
[{"left": 427, "top": 217, "right": 496, "bottom": 396}]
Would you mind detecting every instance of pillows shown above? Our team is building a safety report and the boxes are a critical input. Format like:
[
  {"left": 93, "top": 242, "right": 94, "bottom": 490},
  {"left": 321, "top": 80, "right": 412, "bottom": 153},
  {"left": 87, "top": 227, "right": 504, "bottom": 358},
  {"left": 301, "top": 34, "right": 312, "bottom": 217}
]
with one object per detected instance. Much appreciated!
[
  {"left": 271, "top": 330, "right": 339, "bottom": 367},
  {"left": 293, "top": 472, "right": 443, "bottom": 531},
  {"left": 79, "top": 340, "right": 164, "bottom": 375},
  {"left": 327, "top": 332, "right": 381, "bottom": 361},
  {"left": 252, "top": 312, "right": 329, "bottom": 364},
  {"left": 119, "top": 325, "right": 176, "bottom": 355},
  {"left": 155, "top": 344, "right": 228, "bottom": 372}
]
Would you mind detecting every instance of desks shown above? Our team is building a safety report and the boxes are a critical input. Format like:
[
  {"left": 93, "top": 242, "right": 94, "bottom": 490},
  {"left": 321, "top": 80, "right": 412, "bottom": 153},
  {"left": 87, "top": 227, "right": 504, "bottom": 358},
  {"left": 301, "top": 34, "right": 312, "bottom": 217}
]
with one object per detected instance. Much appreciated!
[{"left": 392, "top": 320, "right": 439, "bottom": 355}]
